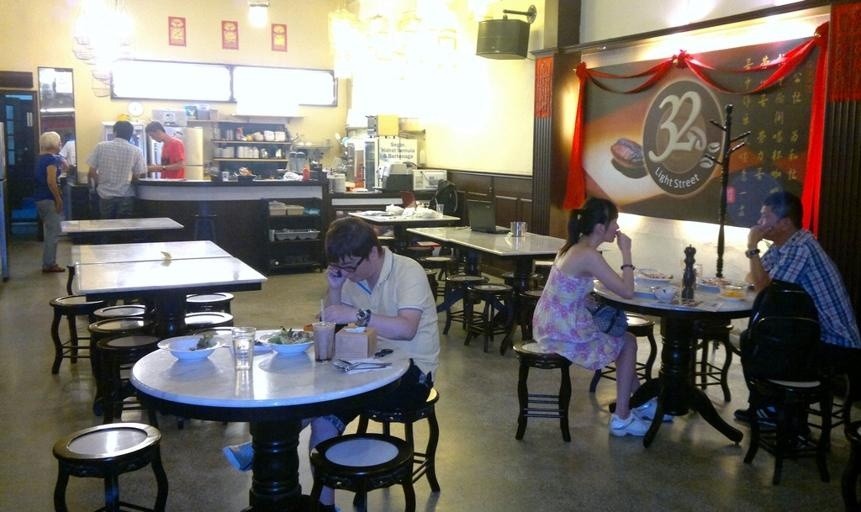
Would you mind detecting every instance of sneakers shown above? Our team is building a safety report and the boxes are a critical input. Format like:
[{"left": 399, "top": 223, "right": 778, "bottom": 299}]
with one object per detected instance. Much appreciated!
[
  {"left": 42, "top": 263, "right": 65, "bottom": 272},
  {"left": 633, "top": 396, "right": 674, "bottom": 421},
  {"left": 759, "top": 428, "right": 812, "bottom": 452},
  {"left": 223, "top": 440, "right": 255, "bottom": 471},
  {"left": 735, "top": 406, "right": 785, "bottom": 427},
  {"left": 610, "top": 409, "right": 653, "bottom": 437}
]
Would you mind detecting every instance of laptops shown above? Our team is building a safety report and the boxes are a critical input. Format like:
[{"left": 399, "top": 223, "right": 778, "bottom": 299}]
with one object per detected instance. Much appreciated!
[{"left": 468, "top": 200, "right": 511, "bottom": 234}]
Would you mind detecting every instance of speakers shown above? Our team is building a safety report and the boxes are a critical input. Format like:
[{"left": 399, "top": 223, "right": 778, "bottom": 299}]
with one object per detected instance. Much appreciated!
[{"left": 476, "top": 15, "right": 531, "bottom": 61}]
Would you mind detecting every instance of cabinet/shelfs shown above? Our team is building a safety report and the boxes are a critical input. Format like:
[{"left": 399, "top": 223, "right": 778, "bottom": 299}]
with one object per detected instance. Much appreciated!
[{"left": 208, "top": 121, "right": 295, "bottom": 175}]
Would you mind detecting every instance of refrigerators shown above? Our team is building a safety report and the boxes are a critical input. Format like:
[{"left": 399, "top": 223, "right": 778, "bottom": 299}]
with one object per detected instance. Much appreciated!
[{"left": 148, "top": 127, "right": 214, "bottom": 180}]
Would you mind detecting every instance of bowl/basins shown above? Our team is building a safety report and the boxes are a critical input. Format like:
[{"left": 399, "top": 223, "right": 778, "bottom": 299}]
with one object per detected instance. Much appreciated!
[
  {"left": 652, "top": 286, "right": 676, "bottom": 303},
  {"left": 157, "top": 335, "right": 224, "bottom": 362},
  {"left": 259, "top": 331, "right": 314, "bottom": 356}
]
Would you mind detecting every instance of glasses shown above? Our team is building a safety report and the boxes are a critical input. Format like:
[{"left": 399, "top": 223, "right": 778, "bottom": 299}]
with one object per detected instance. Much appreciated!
[{"left": 326, "top": 257, "right": 365, "bottom": 272}]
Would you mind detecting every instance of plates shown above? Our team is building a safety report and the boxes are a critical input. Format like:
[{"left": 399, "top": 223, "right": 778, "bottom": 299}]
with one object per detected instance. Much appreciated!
[
  {"left": 234, "top": 344, "right": 272, "bottom": 353},
  {"left": 637, "top": 275, "right": 673, "bottom": 282},
  {"left": 214, "top": 147, "right": 235, "bottom": 159},
  {"left": 701, "top": 282, "right": 732, "bottom": 288},
  {"left": 717, "top": 295, "right": 748, "bottom": 301},
  {"left": 264, "top": 130, "right": 286, "bottom": 142}
]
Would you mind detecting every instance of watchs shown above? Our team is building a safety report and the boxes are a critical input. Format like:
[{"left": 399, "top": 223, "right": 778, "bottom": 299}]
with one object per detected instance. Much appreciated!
[{"left": 357, "top": 309, "right": 370, "bottom": 326}]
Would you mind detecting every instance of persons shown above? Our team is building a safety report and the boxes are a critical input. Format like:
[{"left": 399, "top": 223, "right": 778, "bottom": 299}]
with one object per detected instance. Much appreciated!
[
  {"left": 85, "top": 120, "right": 144, "bottom": 219},
  {"left": 732, "top": 190, "right": 860, "bottom": 428},
  {"left": 222, "top": 216, "right": 442, "bottom": 512},
  {"left": 33, "top": 131, "right": 66, "bottom": 273},
  {"left": 145, "top": 121, "right": 185, "bottom": 178},
  {"left": 55, "top": 139, "right": 75, "bottom": 185},
  {"left": 529, "top": 197, "right": 674, "bottom": 440}
]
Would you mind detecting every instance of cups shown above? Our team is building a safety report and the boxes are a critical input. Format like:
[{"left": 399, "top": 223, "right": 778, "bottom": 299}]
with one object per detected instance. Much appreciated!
[
  {"left": 511, "top": 221, "right": 526, "bottom": 237},
  {"left": 436, "top": 203, "right": 444, "bottom": 217},
  {"left": 231, "top": 325, "right": 255, "bottom": 369},
  {"left": 311, "top": 320, "right": 335, "bottom": 362}
]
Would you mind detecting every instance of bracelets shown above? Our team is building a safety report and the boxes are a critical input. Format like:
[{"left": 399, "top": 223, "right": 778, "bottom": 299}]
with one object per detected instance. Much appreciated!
[
  {"left": 620, "top": 264, "right": 635, "bottom": 270},
  {"left": 162, "top": 166, "right": 165, "bottom": 170},
  {"left": 744, "top": 249, "right": 760, "bottom": 258}
]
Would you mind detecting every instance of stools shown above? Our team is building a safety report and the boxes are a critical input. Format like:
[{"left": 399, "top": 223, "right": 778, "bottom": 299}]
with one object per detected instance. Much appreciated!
[
  {"left": 51, "top": 420, "right": 168, "bottom": 512},
  {"left": 744, "top": 358, "right": 858, "bottom": 512},
  {"left": 48, "top": 289, "right": 103, "bottom": 378}
]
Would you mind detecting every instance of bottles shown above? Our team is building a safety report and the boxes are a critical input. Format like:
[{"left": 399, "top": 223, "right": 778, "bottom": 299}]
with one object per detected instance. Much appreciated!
[
  {"left": 303, "top": 164, "right": 310, "bottom": 180},
  {"left": 694, "top": 263, "right": 704, "bottom": 286}
]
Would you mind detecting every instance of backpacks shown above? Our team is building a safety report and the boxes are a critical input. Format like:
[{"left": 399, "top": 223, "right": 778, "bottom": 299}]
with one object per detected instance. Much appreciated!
[{"left": 747, "top": 281, "right": 824, "bottom": 383}]
